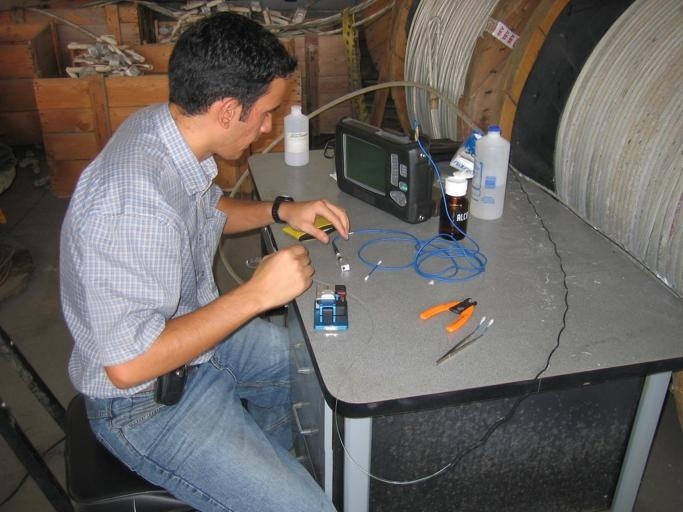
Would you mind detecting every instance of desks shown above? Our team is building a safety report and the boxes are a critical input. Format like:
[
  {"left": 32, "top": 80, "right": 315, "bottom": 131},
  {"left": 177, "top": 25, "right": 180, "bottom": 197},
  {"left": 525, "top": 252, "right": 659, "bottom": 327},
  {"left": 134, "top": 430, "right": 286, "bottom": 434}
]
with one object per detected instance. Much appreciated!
[{"left": 245, "top": 149, "right": 683, "bottom": 511}]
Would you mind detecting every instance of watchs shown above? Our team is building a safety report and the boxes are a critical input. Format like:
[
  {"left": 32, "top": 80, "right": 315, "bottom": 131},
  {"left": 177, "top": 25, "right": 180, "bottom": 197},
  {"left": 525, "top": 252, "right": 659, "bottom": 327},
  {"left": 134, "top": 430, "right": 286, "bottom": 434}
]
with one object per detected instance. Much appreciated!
[{"left": 270, "top": 192, "right": 295, "bottom": 225}]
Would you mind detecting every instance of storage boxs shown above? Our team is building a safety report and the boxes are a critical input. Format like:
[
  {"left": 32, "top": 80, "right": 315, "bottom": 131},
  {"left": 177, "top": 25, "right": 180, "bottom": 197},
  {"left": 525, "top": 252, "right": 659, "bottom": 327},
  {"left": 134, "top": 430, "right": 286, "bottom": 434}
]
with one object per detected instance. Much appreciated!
[{"left": 0, "top": 0, "right": 360, "bottom": 198}]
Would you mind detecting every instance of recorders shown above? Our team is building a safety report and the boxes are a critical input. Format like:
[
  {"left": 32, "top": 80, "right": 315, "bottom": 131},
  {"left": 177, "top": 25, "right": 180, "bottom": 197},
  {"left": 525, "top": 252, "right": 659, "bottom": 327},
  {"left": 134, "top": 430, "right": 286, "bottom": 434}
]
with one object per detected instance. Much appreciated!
[{"left": 335, "top": 116, "right": 433, "bottom": 224}]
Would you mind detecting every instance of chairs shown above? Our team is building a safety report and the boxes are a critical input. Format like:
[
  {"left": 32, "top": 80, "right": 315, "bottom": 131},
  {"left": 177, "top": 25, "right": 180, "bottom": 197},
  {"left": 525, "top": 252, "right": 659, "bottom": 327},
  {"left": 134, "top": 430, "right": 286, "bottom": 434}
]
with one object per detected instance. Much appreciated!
[{"left": 0, "top": 323, "right": 248, "bottom": 512}]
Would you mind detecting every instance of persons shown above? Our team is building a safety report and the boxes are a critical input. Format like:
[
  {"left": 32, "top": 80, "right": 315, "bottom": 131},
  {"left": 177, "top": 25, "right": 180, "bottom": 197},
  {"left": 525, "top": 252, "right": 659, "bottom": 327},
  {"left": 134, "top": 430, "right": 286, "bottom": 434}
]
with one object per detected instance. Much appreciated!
[{"left": 56, "top": 10, "right": 352, "bottom": 512}]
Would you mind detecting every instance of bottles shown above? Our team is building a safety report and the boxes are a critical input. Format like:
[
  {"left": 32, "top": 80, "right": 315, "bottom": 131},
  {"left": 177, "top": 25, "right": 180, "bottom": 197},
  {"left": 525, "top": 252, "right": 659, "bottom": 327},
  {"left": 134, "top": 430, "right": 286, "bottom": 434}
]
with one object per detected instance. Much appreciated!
[
  {"left": 470, "top": 126, "right": 510, "bottom": 220},
  {"left": 284, "top": 105, "right": 309, "bottom": 167},
  {"left": 439, "top": 176, "right": 468, "bottom": 241}
]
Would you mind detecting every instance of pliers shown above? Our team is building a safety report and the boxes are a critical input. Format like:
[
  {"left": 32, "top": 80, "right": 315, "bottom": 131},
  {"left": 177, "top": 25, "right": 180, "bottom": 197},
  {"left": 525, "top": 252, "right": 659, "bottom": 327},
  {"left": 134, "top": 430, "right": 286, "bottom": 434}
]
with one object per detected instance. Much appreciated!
[{"left": 420, "top": 298, "right": 477, "bottom": 333}]
